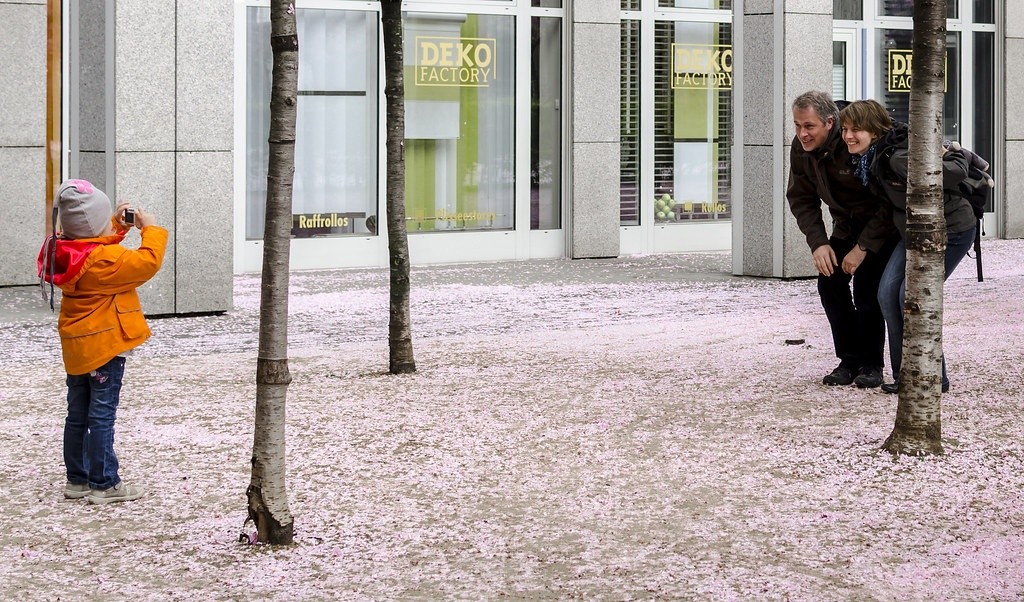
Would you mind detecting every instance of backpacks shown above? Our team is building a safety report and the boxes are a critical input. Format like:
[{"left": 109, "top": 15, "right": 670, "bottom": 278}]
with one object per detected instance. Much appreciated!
[{"left": 880, "top": 139, "right": 989, "bottom": 218}]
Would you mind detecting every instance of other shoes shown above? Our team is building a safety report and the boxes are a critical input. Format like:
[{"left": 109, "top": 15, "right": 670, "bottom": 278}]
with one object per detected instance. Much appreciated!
[
  {"left": 881, "top": 380, "right": 900, "bottom": 394},
  {"left": 942, "top": 382, "right": 949, "bottom": 393},
  {"left": 88, "top": 482, "right": 145, "bottom": 505},
  {"left": 64, "top": 481, "right": 91, "bottom": 498}
]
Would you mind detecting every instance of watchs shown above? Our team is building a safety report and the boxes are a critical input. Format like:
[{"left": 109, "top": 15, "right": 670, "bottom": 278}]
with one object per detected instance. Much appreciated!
[{"left": 858, "top": 244, "right": 869, "bottom": 253}]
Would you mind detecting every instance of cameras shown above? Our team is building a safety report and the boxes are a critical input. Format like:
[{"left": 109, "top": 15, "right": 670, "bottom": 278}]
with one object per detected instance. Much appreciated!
[{"left": 124, "top": 208, "right": 135, "bottom": 225}]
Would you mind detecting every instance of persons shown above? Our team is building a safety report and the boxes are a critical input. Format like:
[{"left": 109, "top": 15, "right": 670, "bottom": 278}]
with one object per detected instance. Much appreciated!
[
  {"left": 838, "top": 100, "right": 979, "bottom": 392},
  {"left": 786, "top": 92, "right": 898, "bottom": 388},
  {"left": 37, "top": 180, "right": 169, "bottom": 505}
]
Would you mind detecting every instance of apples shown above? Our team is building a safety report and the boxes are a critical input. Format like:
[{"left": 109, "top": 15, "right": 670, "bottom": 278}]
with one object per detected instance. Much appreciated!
[{"left": 654, "top": 193, "right": 676, "bottom": 219}]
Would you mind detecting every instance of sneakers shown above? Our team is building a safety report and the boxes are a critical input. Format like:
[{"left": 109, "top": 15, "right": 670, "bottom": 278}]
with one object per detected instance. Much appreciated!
[
  {"left": 855, "top": 363, "right": 884, "bottom": 388},
  {"left": 822, "top": 357, "right": 861, "bottom": 386}
]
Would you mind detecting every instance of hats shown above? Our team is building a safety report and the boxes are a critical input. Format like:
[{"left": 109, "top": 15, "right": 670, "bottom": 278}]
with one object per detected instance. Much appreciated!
[{"left": 40, "top": 179, "right": 111, "bottom": 314}]
